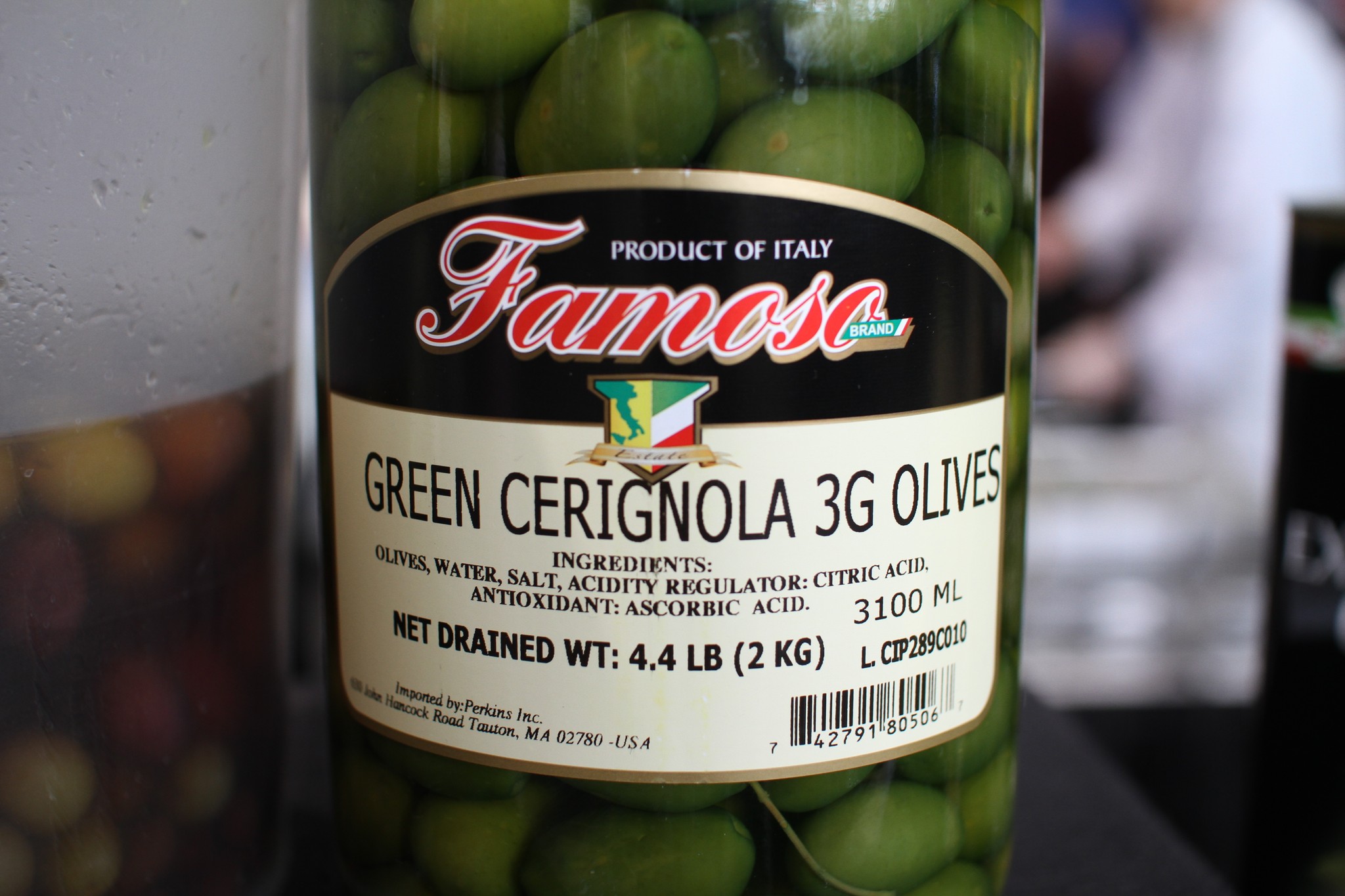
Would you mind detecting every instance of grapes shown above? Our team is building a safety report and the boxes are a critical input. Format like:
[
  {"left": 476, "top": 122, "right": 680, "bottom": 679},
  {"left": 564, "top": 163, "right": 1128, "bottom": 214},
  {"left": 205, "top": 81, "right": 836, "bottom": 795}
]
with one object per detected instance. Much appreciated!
[{"left": 310, "top": 0, "right": 1044, "bottom": 896}]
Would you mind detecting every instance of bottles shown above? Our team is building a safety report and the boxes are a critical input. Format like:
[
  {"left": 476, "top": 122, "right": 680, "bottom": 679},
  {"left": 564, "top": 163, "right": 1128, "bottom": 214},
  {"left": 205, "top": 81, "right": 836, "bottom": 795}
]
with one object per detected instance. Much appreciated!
[{"left": 306, "top": 0, "right": 1046, "bottom": 896}]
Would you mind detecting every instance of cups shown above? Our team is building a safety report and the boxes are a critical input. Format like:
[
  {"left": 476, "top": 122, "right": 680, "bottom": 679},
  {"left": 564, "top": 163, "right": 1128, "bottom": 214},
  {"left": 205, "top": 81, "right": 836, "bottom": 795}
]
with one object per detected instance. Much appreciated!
[
  {"left": 0, "top": 0, "right": 306, "bottom": 896},
  {"left": 1017, "top": 0, "right": 1294, "bottom": 707}
]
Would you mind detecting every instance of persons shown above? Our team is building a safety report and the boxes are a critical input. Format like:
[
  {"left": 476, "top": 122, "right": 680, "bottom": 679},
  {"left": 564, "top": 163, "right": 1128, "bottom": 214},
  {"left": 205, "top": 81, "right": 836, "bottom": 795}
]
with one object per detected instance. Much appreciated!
[{"left": 1036, "top": 0, "right": 1345, "bottom": 475}]
